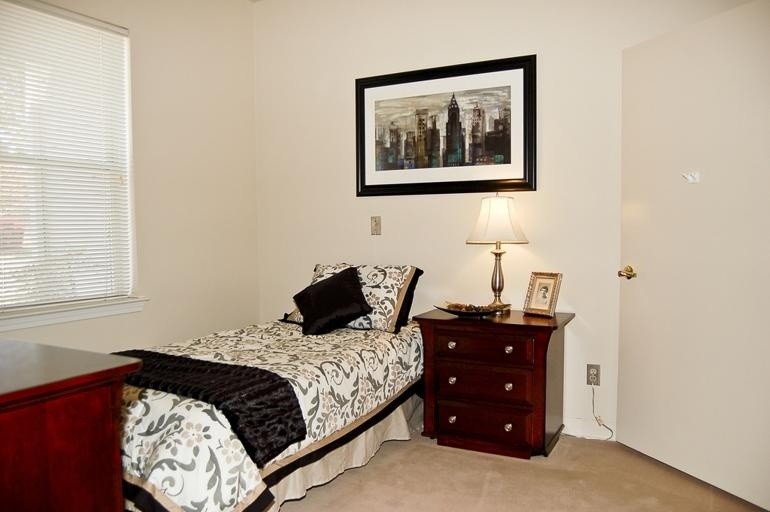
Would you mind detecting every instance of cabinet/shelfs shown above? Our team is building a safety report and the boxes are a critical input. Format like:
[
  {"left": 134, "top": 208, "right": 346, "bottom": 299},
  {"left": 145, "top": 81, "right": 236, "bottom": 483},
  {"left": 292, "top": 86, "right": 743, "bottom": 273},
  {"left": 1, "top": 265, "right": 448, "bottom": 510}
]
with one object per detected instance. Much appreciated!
[{"left": 1, "top": 338, "right": 143, "bottom": 512}]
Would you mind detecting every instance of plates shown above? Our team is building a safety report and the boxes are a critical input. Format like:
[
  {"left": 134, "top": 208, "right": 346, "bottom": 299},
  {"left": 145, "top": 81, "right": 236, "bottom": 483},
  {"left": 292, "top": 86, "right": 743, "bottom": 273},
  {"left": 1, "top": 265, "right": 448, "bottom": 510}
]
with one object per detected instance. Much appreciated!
[{"left": 435, "top": 303, "right": 512, "bottom": 318}]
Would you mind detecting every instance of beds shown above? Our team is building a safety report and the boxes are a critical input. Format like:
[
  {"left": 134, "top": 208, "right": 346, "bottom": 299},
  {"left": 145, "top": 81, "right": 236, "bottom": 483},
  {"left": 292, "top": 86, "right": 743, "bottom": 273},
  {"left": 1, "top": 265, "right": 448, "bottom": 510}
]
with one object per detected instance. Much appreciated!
[{"left": 111, "top": 319, "right": 424, "bottom": 512}]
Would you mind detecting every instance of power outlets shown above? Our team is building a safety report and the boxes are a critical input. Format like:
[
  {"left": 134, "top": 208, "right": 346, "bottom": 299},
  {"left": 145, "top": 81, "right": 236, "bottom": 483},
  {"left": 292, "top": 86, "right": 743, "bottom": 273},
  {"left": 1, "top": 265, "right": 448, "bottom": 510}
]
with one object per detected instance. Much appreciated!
[{"left": 586, "top": 364, "right": 600, "bottom": 386}]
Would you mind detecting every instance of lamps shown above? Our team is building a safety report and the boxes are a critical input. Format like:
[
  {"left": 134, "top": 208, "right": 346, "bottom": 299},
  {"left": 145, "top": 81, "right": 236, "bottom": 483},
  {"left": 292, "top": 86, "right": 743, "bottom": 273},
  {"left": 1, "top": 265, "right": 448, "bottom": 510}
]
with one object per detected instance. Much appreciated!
[{"left": 466, "top": 195, "right": 529, "bottom": 310}]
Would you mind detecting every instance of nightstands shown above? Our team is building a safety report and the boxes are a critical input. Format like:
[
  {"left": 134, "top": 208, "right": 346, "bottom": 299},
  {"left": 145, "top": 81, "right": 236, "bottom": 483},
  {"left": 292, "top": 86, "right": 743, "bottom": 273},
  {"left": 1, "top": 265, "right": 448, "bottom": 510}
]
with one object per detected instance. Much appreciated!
[{"left": 412, "top": 307, "right": 575, "bottom": 460}]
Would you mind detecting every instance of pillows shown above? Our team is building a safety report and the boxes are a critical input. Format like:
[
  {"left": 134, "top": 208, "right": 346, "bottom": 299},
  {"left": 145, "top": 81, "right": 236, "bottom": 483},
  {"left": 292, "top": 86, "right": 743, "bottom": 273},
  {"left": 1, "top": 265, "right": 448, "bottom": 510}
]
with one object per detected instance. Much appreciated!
[
  {"left": 292, "top": 267, "right": 373, "bottom": 335},
  {"left": 278, "top": 263, "right": 424, "bottom": 335}
]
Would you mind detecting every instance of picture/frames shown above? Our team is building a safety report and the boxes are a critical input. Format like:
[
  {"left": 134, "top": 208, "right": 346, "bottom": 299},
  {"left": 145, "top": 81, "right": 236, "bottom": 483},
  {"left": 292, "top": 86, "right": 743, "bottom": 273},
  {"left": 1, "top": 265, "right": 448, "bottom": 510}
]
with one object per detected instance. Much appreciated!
[
  {"left": 523, "top": 271, "right": 563, "bottom": 318},
  {"left": 355, "top": 54, "right": 538, "bottom": 198}
]
